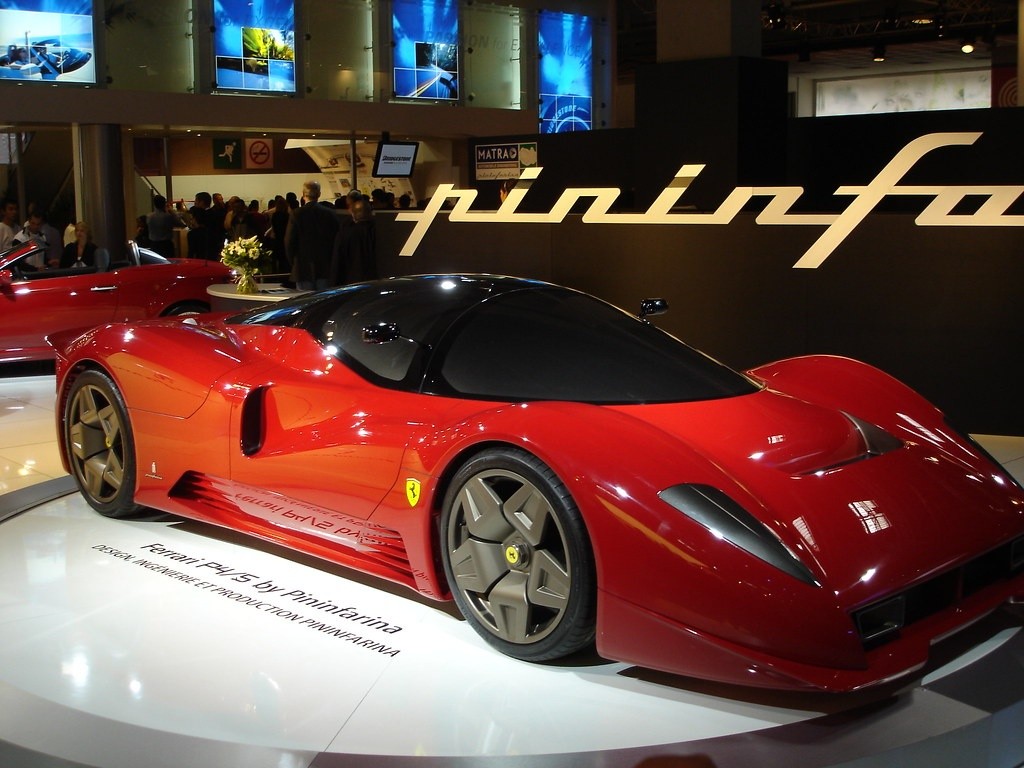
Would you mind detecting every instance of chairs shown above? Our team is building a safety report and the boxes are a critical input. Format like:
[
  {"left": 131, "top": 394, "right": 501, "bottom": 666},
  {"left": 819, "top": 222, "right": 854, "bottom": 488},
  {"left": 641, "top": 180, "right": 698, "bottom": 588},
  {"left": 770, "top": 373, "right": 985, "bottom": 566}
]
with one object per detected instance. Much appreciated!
[{"left": 96, "top": 248, "right": 110, "bottom": 273}]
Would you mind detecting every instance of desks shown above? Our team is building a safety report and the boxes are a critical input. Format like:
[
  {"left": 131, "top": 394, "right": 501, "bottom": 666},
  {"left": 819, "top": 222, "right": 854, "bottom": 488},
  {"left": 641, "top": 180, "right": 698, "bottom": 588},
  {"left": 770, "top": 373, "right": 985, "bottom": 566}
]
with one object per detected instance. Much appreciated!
[{"left": 207, "top": 284, "right": 314, "bottom": 303}]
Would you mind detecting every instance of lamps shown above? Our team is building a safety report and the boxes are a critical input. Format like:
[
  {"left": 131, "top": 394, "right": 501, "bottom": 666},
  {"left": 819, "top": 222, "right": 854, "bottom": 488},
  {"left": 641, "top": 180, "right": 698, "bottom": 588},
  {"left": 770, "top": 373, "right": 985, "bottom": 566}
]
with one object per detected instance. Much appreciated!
[
  {"left": 304, "top": 33, "right": 311, "bottom": 41},
  {"left": 391, "top": 91, "right": 396, "bottom": 98},
  {"left": 390, "top": 40, "right": 395, "bottom": 48},
  {"left": 208, "top": 25, "right": 216, "bottom": 33},
  {"left": 106, "top": 76, "right": 114, "bottom": 83},
  {"left": 538, "top": 118, "right": 543, "bottom": 123},
  {"left": 306, "top": 86, "right": 313, "bottom": 94},
  {"left": 211, "top": 81, "right": 219, "bottom": 88}
]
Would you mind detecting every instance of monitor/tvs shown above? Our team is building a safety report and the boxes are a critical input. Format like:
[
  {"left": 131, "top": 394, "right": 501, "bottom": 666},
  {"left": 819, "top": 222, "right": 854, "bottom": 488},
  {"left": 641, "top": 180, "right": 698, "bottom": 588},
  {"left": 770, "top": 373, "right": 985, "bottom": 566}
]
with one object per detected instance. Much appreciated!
[{"left": 372, "top": 140, "right": 419, "bottom": 178}]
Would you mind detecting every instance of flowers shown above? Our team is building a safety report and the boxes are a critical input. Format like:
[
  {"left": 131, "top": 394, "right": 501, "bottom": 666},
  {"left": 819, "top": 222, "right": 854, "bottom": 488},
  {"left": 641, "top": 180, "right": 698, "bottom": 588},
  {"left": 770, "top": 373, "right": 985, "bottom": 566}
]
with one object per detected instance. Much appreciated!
[{"left": 220, "top": 236, "right": 272, "bottom": 293}]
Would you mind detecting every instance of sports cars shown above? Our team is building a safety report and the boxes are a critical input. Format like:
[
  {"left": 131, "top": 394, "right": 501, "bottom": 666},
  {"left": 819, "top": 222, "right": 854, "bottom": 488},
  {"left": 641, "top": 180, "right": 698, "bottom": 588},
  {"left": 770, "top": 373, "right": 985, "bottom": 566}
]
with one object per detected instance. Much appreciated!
[
  {"left": 41, "top": 271, "right": 1024, "bottom": 698},
  {"left": 0, "top": 237, "right": 258, "bottom": 368}
]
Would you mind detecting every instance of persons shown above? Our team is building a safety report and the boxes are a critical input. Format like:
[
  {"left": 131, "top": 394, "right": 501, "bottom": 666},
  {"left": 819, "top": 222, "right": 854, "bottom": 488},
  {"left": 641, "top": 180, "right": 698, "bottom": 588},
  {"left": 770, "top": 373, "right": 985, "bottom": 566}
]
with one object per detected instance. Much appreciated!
[
  {"left": 285, "top": 179, "right": 341, "bottom": 291},
  {"left": 331, "top": 198, "right": 376, "bottom": 283},
  {"left": 0, "top": 201, "right": 22, "bottom": 253},
  {"left": 58, "top": 221, "right": 97, "bottom": 268},
  {"left": 11, "top": 211, "right": 46, "bottom": 266},
  {"left": 134, "top": 189, "right": 412, "bottom": 276},
  {"left": 24, "top": 204, "right": 61, "bottom": 245}
]
